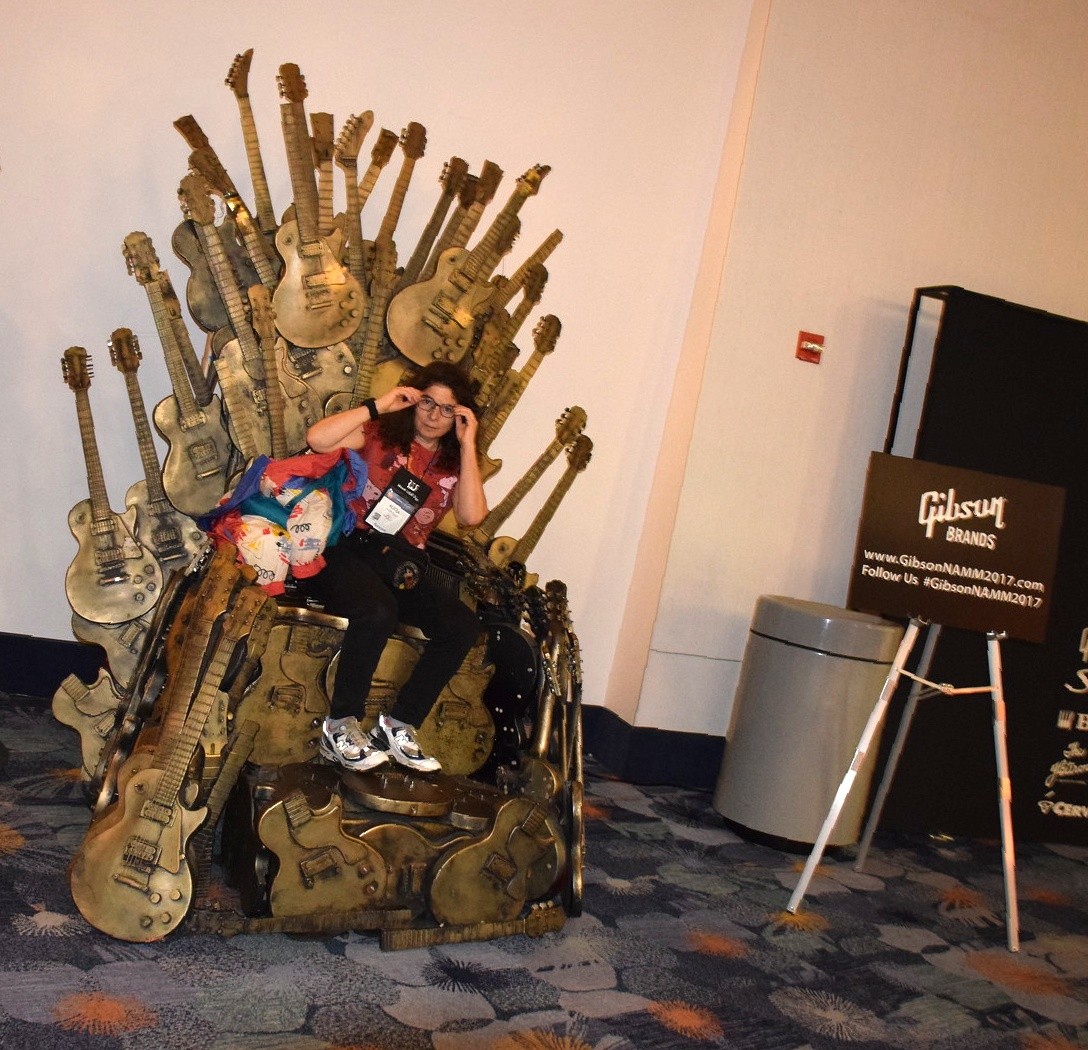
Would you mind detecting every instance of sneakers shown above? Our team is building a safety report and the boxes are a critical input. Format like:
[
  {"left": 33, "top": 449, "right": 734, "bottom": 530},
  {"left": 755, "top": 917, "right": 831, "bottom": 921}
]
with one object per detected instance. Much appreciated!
[
  {"left": 320, "top": 717, "right": 388, "bottom": 773},
  {"left": 367, "top": 712, "right": 443, "bottom": 771}
]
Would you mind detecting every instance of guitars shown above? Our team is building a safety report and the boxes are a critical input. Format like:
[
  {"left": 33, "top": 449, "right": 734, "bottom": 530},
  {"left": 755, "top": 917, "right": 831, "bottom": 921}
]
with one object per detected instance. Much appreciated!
[{"left": 52, "top": 46, "right": 594, "bottom": 945}]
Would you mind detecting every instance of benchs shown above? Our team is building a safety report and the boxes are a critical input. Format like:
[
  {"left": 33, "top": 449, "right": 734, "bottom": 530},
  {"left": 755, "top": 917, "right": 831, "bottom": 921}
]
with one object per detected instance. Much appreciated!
[{"left": 274, "top": 594, "right": 488, "bottom": 648}]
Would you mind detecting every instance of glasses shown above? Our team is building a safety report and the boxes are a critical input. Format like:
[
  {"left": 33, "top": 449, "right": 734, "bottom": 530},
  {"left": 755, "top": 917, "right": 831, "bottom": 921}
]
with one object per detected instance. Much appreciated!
[{"left": 416, "top": 396, "right": 456, "bottom": 418}]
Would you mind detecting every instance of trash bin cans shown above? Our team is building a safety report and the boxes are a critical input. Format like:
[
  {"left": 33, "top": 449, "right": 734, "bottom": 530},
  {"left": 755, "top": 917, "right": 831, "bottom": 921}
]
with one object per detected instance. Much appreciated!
[{"left": 709, "top": 593, "right": 905, "bottom": 861}]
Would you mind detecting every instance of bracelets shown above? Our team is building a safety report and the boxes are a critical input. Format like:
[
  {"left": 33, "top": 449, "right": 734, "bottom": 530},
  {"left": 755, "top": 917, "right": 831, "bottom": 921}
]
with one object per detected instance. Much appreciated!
[{"left": 361, "top": 398, "right": 379, "bottom": 420}]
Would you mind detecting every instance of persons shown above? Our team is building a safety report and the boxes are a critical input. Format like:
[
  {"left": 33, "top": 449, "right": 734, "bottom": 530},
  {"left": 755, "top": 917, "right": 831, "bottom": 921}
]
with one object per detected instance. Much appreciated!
[{"left": 306, "top": 361, "right": 488, "bottom": 776}]
